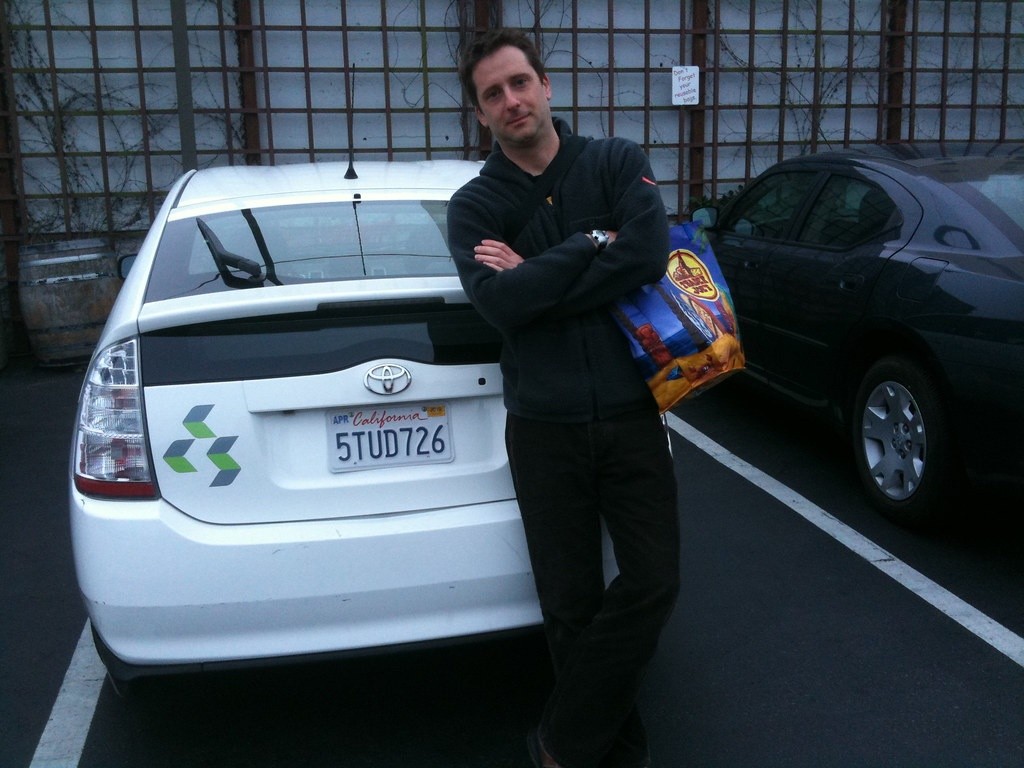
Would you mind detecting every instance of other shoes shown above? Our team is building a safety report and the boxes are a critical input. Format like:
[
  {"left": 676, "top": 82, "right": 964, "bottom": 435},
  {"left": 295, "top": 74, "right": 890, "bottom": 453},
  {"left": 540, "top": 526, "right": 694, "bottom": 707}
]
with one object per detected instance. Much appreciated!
[
  {"left": 526, "top": 723, "right": 563, "bottom": 768},
  {"left": 600, "top": 704, "right": 653, "bottom": 768}
]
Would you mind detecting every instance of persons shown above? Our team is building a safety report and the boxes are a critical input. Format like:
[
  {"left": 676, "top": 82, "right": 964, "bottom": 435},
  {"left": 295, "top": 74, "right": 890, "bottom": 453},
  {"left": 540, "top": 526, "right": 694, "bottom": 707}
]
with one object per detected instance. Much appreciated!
[{"left": 445, "top": 26, "right": 683, "bottom": 768}]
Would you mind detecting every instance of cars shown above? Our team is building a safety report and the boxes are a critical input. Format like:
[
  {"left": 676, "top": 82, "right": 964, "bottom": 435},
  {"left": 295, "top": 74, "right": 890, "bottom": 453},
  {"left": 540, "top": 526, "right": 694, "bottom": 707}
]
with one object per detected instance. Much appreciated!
[
  {"left": 67, "top": 158, "right": 615, "bottom": 698},
  {"left": 691, "top": 142, "right": 1023, "bottom": 531}
]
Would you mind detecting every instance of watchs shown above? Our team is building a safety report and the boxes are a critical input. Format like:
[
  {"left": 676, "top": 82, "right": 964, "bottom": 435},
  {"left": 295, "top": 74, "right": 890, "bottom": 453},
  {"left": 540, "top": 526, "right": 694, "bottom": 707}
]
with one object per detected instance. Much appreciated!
[{"left": 585, "top": 229, "right": 609, "bottom": 254}]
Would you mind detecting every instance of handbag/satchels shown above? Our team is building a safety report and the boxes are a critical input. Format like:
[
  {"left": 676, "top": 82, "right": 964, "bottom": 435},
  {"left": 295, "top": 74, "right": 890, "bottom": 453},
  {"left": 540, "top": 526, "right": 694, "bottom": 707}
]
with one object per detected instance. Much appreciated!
[{"left": 604, "top": 218, "right": 748, "bottom": 413}]
